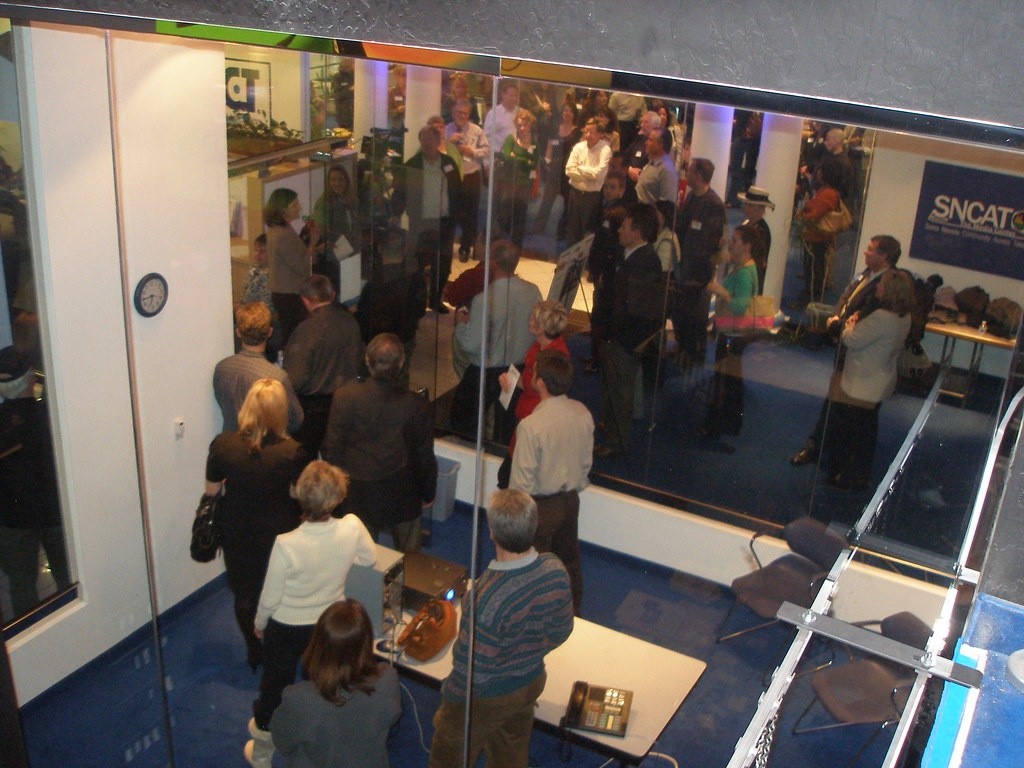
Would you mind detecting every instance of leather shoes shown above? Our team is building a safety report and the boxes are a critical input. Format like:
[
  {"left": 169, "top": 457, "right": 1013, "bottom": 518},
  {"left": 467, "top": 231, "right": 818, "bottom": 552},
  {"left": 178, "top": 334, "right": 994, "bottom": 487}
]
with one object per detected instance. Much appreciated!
[
  {"left": 827, "top": 454, "right": 851, "bottom": 474},
  {"left": 791, "top": 449, "right": 816, "bottom": 466}
]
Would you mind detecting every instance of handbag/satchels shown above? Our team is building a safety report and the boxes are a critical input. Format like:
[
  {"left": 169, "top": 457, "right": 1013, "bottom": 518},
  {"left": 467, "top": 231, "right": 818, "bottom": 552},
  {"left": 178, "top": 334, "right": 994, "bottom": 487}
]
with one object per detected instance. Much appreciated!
[
  {"left": 814, "top": 188, "right": 852, "bottom": 234},
  {"left": 397, "top": 597, "right": 457, "bottom": 661},
  {"left": 901, "top": 344, "right": 932, "bottom": 381},
  {"left": 527, "top": 144, "right": 539, "bottom": 196},
  {"left": 714, "top": 268, "right": 775, "bottom": 335},
  {"left": 653, "top": 239, "right": 693, "bottom": 311},
  {"left": 192, "top": 482, "right": 222, "bottom": 563}
]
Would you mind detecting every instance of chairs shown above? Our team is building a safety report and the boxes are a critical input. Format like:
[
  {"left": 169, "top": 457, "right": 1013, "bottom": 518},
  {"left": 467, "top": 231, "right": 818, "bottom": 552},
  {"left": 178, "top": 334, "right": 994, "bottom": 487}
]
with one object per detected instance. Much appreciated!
[
  {"left": 716, "top": 516, "right": 844, "bottom": 689},
  {"left": 792, "top": 611, "right": 934, "bottom": 768}
]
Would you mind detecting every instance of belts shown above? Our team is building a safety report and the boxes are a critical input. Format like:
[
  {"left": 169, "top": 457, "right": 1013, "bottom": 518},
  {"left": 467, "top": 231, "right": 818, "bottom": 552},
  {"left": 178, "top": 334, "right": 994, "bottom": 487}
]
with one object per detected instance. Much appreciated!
[{"left": 572, "top": 187, "right": 598, "bottom": 196}]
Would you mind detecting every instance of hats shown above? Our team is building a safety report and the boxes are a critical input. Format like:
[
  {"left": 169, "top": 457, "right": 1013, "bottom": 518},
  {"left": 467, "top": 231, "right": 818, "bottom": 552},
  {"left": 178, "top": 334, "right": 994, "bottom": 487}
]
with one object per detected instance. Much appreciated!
[
  {"left": 1, "top": 339, "right": 38, "bottom": 382},
  {"left": 738, "top": 186, "right": 775, "bottom": 210}
]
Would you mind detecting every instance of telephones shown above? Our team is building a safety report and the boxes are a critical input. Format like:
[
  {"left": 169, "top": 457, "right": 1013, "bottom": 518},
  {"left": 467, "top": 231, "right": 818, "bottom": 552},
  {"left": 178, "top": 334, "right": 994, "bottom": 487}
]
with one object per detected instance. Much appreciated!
[{"left": 563, "top": 680, "right": 633, "bottom": 738}]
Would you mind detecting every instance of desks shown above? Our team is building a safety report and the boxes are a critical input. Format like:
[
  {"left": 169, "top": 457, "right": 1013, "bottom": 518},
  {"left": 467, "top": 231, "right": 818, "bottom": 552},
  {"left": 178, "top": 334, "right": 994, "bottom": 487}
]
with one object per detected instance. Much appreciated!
[
  {"left": 373, "top": 578, "right": 709, "bottom": 768},
  {"left": 925, "top": 321, "right": 1017, "bottom": 408}
]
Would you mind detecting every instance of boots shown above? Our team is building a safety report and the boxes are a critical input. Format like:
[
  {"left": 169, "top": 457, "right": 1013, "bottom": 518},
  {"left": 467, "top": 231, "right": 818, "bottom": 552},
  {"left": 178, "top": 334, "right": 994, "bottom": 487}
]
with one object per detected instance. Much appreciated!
[{"left": 243, "top": 717, "right": 278, "bottom": 768}]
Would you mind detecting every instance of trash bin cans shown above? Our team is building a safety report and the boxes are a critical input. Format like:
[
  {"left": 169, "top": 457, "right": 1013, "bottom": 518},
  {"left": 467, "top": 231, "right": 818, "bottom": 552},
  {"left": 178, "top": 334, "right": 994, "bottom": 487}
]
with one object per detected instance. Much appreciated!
[{"left": 423, "top": 455, "right": 461, "bottom": 519}]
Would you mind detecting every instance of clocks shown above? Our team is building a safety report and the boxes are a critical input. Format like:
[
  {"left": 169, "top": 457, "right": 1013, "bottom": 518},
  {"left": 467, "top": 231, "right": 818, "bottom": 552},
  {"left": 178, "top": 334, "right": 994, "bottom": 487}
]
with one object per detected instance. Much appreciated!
[{"left": 134, "top": 273, "right": 168, "bottom": 317}]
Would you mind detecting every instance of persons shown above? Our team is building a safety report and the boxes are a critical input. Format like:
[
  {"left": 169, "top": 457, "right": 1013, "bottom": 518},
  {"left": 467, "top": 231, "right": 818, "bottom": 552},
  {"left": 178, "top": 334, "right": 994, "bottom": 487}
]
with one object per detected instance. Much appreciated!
[
  {"left": 428, "top": 487, "right": 575, "bottom": 768},
  {"left": 268, "top": 599, "right": 401, "bottom": 768},
  {"left": 0, "top": 344, "right": 71, "bottom": 619},
  {"left": 507, "top": 352, "right": 596, "bottom": 621},
  {"left": 204, "top": 376, "right": 310, "bottom": 670},
  {"left": 0, "top": 163, "right": 25, "bottom": 319},
  {"left": 213, "top": 55, "right": 919, "bottom": 486},
  {"left": 244, "top": 460, "right": 377, "bottom": 768},
  {"left": 318, "top": 335, "right": 439, "bottom": 554}
]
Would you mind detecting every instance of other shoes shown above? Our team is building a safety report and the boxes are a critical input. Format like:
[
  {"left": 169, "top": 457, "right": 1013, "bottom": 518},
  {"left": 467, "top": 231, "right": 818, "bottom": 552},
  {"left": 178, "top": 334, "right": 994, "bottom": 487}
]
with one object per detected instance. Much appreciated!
[
  {"left": 679, "top": 423, "right": 720, "bottom": 443},
  {"left": 430, "top": 300, "right": 449, "bottom": 313},
  {"left": 459, "top": 251, "right": 469, "bottom": 262},
  {"left": 593, "top": 443, "right": 615, "bottom": 459}
]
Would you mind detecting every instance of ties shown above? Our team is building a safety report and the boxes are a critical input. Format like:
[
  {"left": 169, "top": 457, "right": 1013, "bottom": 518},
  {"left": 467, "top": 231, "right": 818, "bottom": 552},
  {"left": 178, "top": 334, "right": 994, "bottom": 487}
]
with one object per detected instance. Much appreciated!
[
  {"left": 840, "top": 277, "right": 870, "bottom": 315},
  {"left": 456, "top": 129, "right": 463, "bottom": 147}
]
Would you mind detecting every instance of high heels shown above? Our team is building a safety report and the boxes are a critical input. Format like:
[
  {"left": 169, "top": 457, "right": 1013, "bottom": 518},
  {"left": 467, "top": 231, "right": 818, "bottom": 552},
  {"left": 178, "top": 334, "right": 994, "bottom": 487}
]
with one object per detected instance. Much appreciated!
[
  {"left": 832, "top": 464, "right": 860, "bottom": 491},
  {"left": 850, "top": 459, "right": 865, "bottom": 479}
]
